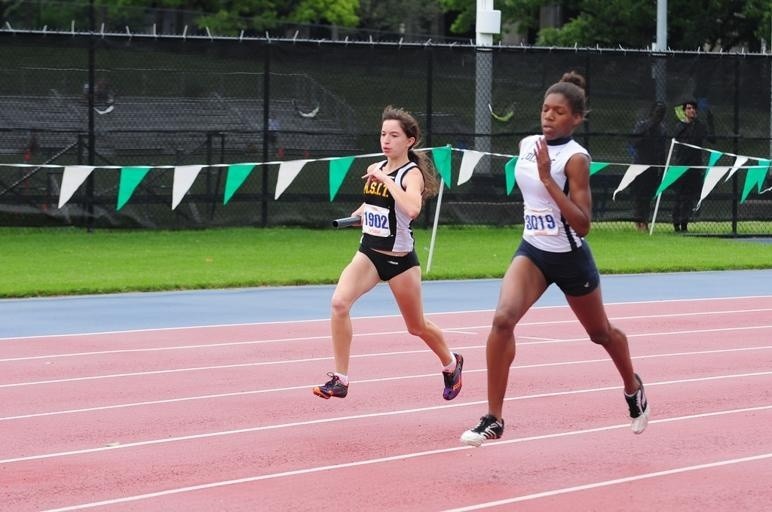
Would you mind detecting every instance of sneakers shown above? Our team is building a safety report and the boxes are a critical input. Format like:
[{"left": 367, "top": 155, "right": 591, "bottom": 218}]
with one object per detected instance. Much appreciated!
[
  {"left": 624, "top": 373, "right": 649, "bottom": 435},
  {"left": 312, "top": 373, "right": 349, "bottom": 400},
  {"left": 460, "top": 414, "right": 505, "bottom": 447},
  {"left": 442, "top": 351, "right": 464, "bottom": 401},
  {"left": 631, "top": 222, "right": 650, "bottom": 234},
  {"left": 674, "top": 224, "right": 692, "bottom": 235}
]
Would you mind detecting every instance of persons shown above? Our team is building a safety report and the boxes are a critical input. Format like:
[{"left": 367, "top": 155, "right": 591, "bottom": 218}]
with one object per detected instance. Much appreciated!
[
  {"left": 458, "top": 70, "right": 650, "bottom": 448},
  {"left": 269, "top": 110, "right": 279, "bottom": 147},
  {"left": 313, "top": 105, "right": 464, "bottom": 400},
  {"left": 630, "top": 99, "right": 716, "bottom": 232}
]
